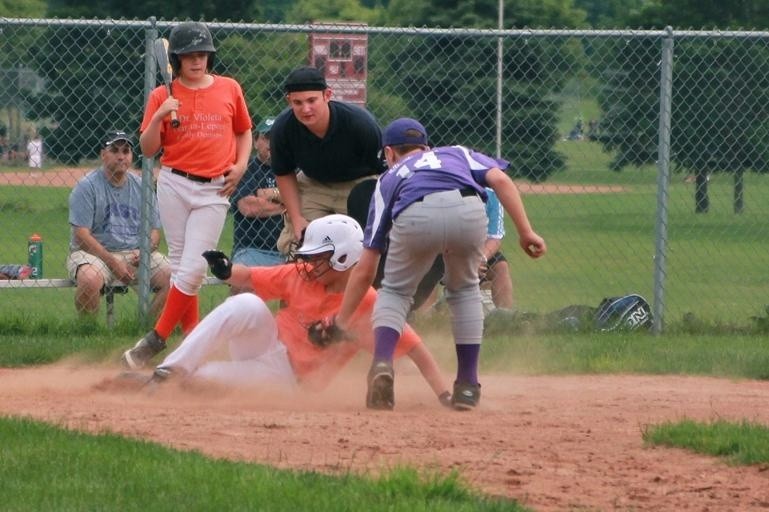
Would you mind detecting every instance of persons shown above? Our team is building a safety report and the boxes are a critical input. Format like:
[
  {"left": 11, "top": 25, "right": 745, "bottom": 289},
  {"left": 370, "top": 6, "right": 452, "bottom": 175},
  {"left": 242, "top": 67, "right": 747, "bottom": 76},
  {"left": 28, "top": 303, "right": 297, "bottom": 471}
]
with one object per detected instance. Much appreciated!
[{"left": 67, "top": 130, "right": 171, "bottom": 334}]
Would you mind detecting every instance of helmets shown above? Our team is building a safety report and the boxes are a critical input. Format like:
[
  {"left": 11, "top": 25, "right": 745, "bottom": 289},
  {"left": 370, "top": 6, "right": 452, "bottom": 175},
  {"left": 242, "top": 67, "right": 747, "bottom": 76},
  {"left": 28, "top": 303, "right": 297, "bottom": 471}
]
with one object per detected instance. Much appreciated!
[{"left": 168, "top": 21, "right": 216, "bottom": 75}]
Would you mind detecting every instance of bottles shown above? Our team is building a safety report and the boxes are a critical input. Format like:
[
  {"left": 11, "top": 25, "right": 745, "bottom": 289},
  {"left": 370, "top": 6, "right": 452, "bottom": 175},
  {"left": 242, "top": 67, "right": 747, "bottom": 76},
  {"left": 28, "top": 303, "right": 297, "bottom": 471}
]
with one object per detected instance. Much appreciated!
[
  {"left": 0, "top": 263, "right": 31, "bottom": 279},
  {"left": 27, "top": 233, "right": 44, "bottom": 278}
]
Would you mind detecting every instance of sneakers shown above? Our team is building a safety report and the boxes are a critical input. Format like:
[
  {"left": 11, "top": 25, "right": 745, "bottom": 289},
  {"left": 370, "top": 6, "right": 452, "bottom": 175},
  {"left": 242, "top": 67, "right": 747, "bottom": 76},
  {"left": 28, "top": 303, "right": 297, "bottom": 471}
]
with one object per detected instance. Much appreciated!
[
  {"left": 451, "top": 377, "right": 481, "bottom": 409},
  {"left": 121, "top": 328, "right": 166, "bottom": 371},
  {"left": 137, "top": 370, "right": 172, "bottom": 399},
  {"left": 365, "top": 363, "right": 395, "bottom": 411}
]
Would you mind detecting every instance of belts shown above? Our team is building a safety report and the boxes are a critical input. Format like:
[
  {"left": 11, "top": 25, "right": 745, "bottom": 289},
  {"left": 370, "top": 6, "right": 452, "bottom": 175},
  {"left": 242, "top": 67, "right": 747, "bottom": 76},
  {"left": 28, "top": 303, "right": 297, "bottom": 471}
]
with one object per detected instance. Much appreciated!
[
  {"left": 415, "top": 189, "right": 478, "bottom": 202},
  {"left": 172, "top": 168, "right": 211, "bottom": 183}
]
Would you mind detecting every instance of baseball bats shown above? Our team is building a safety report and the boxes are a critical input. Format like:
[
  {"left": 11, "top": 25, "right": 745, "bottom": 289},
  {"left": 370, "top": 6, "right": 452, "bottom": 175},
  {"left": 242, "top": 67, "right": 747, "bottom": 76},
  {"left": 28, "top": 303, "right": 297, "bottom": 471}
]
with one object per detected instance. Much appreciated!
[{"left": 156, "top": 38, "right": 182, "bottom": 129}]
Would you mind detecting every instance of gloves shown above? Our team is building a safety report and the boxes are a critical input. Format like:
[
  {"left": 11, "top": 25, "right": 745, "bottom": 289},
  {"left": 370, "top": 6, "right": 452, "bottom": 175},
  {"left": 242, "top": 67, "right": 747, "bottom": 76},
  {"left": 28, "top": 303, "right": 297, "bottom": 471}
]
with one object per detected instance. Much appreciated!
[
  {"left": 202, "top": 249, "right": 232, "bottom": 280},
  {"left": 308, "top": 313, "right": 347, "bottom": 350},
  {"left": 292, "top": 212, "right": 365, "bottom": 282},
  {"left": 438, "top": 391, "right": 452, "bottom": 407}
]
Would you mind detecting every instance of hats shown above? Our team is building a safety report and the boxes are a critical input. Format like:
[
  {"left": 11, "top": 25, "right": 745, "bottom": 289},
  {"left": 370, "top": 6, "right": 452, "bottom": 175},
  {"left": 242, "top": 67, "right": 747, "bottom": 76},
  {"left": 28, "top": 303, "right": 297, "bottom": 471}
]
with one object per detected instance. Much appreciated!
[
  {"left": 254, "top": 115, "right": 278, "bottom": 136},
  {"left": 101, "top": 130, "right": 135, "bottom": 149},
  {"left": 283, "top": 67, "right": 327, "bottom": 93},
  {"left": 379, "top": 117, "right": 428, "bottom": 161}
]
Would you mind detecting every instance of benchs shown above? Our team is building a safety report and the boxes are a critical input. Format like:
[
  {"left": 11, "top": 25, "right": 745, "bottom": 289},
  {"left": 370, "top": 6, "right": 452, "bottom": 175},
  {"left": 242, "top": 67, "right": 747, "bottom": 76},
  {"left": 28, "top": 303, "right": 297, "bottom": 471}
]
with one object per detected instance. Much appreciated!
[{"left": 1, "top": 275, "right": 228, "bottom": 330}]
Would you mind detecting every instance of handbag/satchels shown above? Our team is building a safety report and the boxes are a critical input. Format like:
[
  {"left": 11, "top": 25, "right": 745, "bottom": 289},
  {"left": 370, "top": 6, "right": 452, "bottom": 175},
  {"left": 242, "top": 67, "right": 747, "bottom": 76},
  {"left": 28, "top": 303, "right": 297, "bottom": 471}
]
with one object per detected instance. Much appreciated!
[{"left": 583, "top": 295, "right": 653, "bottom": 335}]
